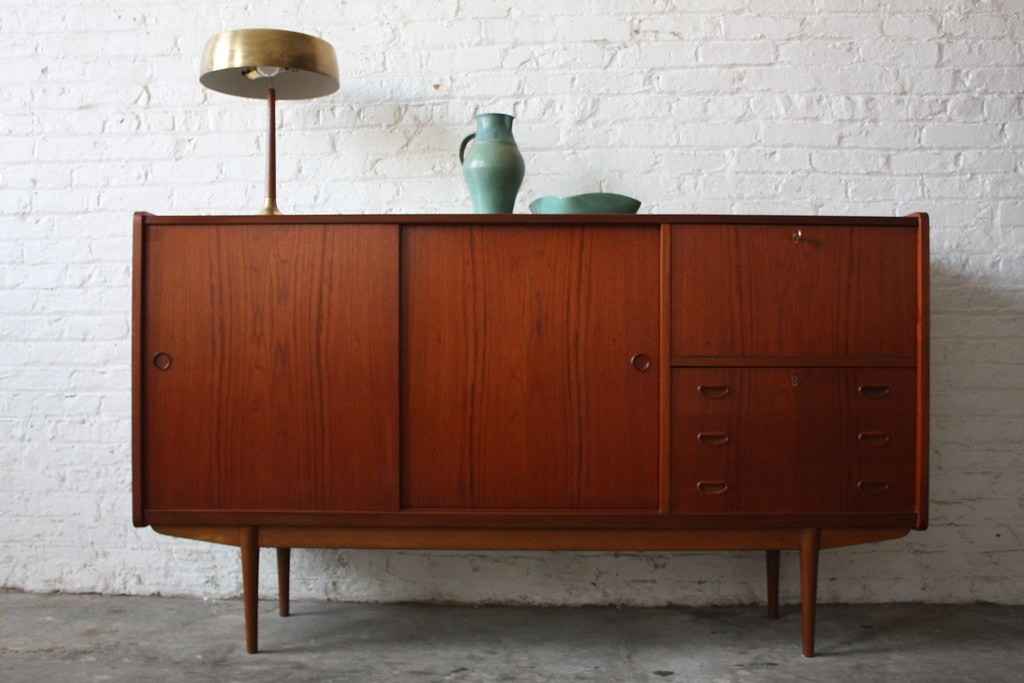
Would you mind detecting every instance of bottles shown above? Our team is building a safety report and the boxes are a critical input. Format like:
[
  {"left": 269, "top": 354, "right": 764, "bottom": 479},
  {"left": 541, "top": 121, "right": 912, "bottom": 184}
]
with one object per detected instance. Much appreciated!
[{"left": 459, "top": 113, "right": 525, "bottom": 214}]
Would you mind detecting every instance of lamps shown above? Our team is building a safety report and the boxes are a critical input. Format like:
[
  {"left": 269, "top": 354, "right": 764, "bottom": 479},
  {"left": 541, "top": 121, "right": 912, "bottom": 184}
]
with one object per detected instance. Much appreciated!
[{"left": 198, "top": 28, "right": 341, "bottom": 215}]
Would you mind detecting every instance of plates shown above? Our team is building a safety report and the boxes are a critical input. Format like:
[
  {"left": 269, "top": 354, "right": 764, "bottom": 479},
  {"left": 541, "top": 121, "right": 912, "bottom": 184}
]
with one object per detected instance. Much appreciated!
[{"left": 528, "top": 192, "right": 641, "bottom": 213}]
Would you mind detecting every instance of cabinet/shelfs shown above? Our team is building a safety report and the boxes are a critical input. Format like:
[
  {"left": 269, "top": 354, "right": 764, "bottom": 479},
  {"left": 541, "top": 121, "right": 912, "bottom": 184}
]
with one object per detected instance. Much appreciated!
[{"left": 131, "top": 211, "right": 930, "bottom": 658}]
